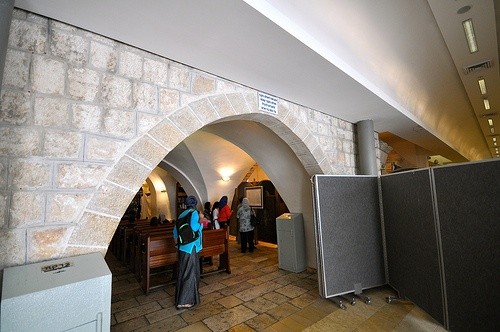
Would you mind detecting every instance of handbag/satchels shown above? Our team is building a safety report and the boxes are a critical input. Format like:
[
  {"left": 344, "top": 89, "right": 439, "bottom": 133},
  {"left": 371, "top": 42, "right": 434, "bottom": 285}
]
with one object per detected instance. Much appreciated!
[
  {"left": 250, "top": 208, "right": 259, "bottom": 227},
  {"left": 219, "top": 219, "right": 230, "bottom": 228}
]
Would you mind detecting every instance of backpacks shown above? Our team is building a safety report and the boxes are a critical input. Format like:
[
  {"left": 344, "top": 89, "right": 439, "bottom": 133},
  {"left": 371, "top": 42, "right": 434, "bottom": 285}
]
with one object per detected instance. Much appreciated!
[{"left": 175, "top": 209, "right": 199, "bottom": 245}]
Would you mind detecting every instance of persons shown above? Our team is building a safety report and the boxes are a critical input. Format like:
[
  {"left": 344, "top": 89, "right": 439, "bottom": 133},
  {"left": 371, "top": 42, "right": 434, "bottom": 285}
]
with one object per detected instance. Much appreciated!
[
  {"left": 219, "top": 196, "right": 233, "bottom": 229},
  {"left": 173, "top": 195, "right": 204, "bottom": 309},
  {"left": 203, "top": 201, "right": 220, "bottom": 230},
  {"left": 236, "top": 198, "right": 256, "bottom": 253}
]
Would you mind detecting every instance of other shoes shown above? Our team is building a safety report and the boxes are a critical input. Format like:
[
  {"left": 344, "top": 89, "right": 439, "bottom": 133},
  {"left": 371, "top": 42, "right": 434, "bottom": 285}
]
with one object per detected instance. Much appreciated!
[{"left": 176, "top": 303, "right": 193, "bottom": 309}]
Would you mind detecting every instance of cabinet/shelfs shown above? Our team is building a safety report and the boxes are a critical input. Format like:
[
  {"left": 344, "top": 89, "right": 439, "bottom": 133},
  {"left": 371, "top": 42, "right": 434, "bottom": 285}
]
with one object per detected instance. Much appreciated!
[{"left": 176, "top": 181, "right": 189, "bottom": 220}]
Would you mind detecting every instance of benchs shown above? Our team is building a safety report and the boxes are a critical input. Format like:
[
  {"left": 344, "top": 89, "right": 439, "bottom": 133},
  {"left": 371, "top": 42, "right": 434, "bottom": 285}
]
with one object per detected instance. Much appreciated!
[{"left": 107, "top": 216, "right": 232, "bottom": 296}]
